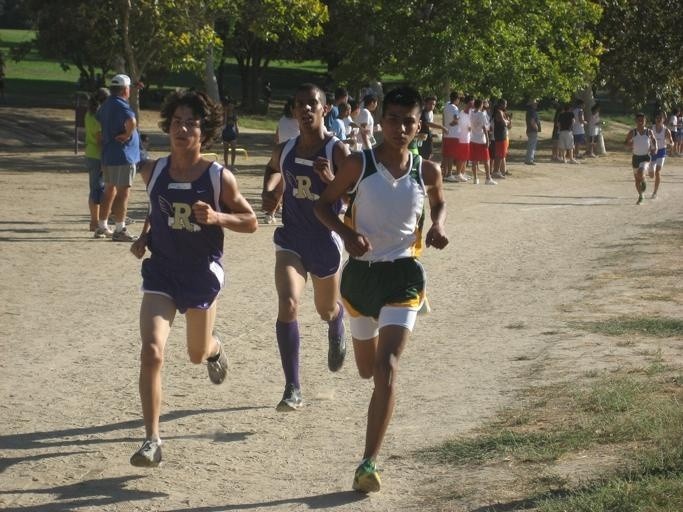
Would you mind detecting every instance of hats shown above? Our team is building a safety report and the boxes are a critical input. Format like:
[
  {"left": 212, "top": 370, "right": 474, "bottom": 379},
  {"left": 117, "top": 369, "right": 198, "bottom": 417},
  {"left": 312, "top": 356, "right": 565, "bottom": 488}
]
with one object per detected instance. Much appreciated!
[{"left": 111, "top": 74, "right": 131, "bottom": 86}]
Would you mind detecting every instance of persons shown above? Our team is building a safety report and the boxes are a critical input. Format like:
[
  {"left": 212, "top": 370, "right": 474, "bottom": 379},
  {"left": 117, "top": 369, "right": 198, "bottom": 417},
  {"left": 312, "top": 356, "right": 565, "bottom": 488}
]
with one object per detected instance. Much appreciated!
[
  {"left": 85, "top": 90, "right": 107, "bottom": 225},
  {"left": 128, "top": 87, "right": 263, "bottom": 466},
  {"left": 674, "top": 108, "right": 682, "bottom": 156},
  {"left": 310, "top": 87, "right": 448, "bottom": 494},
  {"left": 668, "top": 107, "right": 683, "bottom": 158},
  {"left": 552, "top": 100, "right": 602, "bottom": 163},
  {"left": 648, "top": 109, "right": 675, "bottom": 200},
  {"left": 526, "top": 99, "right": 541, "bottom": 164},
  {"left": 277, "top": 88, "right": 513, "bottom": 185},
  {"left": 222, "top": 94, "right": 239, "bottom": 168},
  {"left": 260, "top": 82, "right": 352, "bottom": 413},
  {"left": 96, "top": 72, "right": 143, "bottom": 239},
  {"left": 622, "top": 111, "right": 659, "bottom": 206}
]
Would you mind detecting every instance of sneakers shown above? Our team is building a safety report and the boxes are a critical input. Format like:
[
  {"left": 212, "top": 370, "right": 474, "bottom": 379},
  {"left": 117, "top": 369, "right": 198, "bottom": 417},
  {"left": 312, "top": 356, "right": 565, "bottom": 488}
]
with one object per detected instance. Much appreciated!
[
  {"left": 206, "top": 330, "right": 228, "bottom": 386},
  {"left": 94, "top": 227, "right": 113, "bottom": 238},
  {"left": 130, "top": 441, "right": 162, "bottom": 469},
  {"left": 108, "top": 214, "right": 135, "bottom": 226},
  {"left": 328, "top": 321, "right": 346, "bottom": 372},
  {"left": 351, "top": 457, "right": 380, "bottom": 494},
  {"left": 493, "top": 173, "right": 504, "bottom": 179},
  {"left": 275, "top": 383, "right": 302, "bottom": 413},
  {"left": 111, "top": 227, "right": 140, "bottom": 242},
  {"left": 457, "top": 175, "right": 466, "bottom": 182},
  {"left": 445, "top": 176, "right": 457, "bottom": 182},
  {"left": 485, "top": 179, "right": 497, "bottom": 185},
  {"left": 90, "top": 223, "right": 100, "bottom": 232},
  {"left": 641, "top": 181, "right": 646, "bottom": 192},
  {"left": 505, "top": 170, "right": 512, "bottom": 175},
  {"left": 637, "top": 197, "right": 643, "bottom": 205}
]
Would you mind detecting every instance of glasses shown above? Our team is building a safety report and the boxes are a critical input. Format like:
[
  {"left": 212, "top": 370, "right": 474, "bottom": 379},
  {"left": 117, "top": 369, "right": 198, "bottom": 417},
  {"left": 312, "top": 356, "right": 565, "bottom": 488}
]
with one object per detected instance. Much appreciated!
[{"left": 168, "top": 118, "right": 201, "bottom": 130}]
